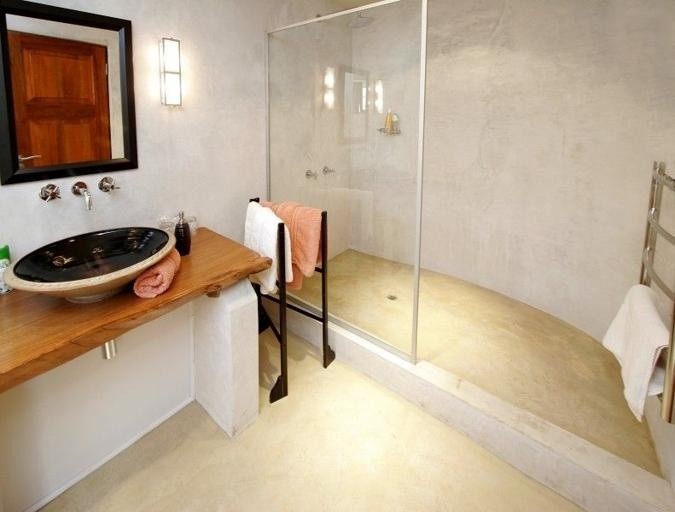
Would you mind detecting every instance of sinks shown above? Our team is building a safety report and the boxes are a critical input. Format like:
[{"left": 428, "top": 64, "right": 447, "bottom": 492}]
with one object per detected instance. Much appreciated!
[{"left": 3, "top": 224, "right": 176, "bottom": 304}]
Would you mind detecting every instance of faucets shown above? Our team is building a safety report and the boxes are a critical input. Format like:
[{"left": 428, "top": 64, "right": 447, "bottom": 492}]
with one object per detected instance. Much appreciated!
[{"left": 79, "top": 186, "right": 93, "bottom": 210}]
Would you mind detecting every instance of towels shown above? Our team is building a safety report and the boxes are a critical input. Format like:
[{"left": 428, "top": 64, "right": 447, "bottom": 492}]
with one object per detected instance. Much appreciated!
[
  {"left": 602, "top": 284, "right": 674, "bottom": 424},
  {"left": 244, "top": 202, "right": 294, "bottom": 297},
  {"left": 259, "top": 201, "right": 329, "bottom": 290},
  {"left": 133, "top": 249, "right": 181, "bottom": 299}
]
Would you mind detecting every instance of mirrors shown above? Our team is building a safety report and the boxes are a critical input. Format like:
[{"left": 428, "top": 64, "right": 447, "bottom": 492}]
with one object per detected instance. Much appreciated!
[{"left": 0, "top": 0, "right": 138, "bottom": 185}]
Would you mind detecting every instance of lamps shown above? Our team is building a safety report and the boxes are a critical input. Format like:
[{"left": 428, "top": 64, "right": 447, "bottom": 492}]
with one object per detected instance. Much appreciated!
[{"left": 160, "top": 38, "right": 183, "bottom": 107}]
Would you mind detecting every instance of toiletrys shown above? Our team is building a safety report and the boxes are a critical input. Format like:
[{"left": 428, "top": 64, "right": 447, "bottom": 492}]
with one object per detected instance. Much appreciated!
[{"left": 0, "top": 244, "right": 13, "bottom": 295}]
